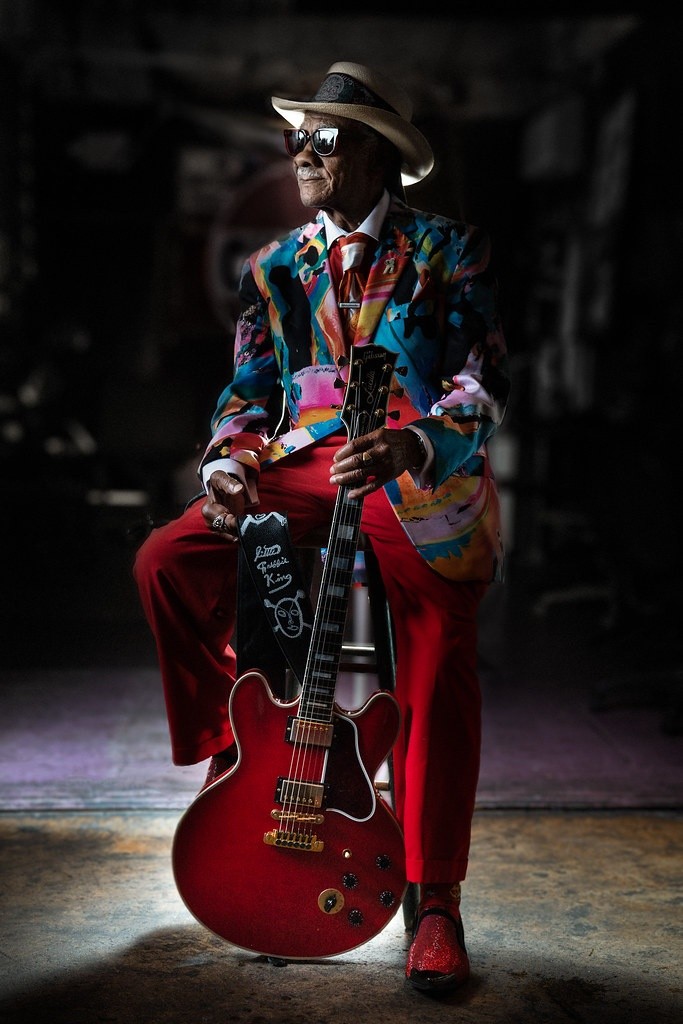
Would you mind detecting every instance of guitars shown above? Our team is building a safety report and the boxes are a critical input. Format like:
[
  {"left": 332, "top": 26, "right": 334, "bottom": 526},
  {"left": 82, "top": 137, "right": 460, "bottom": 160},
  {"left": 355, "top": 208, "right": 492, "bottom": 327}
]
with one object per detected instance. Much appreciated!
[{"left": 171, "top": 341, "right": 411, "bottom": 964}]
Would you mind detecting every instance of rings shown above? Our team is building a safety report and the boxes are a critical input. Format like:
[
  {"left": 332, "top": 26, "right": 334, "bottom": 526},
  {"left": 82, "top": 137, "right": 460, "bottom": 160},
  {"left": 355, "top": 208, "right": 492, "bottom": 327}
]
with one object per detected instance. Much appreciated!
[
  {"left": 212, "top": 511, "right": 231, "bottom": 532},
  {"left": 362, "top": 449, "right": 374, "bottom": 467}
]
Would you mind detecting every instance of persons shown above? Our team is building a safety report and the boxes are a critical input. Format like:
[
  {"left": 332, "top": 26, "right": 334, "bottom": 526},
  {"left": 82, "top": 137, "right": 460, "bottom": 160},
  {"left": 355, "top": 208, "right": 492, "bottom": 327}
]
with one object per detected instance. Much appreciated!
[{"left": 133, "top": 62, "right": 516, "bottom": 994}]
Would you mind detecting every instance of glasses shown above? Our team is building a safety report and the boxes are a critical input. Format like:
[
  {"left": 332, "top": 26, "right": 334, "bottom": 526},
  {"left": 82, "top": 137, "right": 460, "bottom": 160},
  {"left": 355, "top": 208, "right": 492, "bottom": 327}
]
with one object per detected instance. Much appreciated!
[{"left": 283, "top": 127, "right": 359, "bottom": 155}]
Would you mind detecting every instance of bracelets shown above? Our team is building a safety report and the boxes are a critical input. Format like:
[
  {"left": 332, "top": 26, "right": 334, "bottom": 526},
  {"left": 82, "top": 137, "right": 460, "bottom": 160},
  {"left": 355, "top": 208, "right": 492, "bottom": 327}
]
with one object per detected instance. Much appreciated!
[{"left": 404, "top": 428, "right": 427, "bottom": 470}]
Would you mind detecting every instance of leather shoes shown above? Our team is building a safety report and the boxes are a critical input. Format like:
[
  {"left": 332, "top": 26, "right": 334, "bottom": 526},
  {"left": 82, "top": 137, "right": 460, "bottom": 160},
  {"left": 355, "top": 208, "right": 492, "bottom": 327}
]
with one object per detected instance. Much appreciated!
[
  {"left": 202, "top": 751, "right": 236, "bottom": 786},
  {"left": 405, "top": 908, "right": 470, "bottom": 990}
]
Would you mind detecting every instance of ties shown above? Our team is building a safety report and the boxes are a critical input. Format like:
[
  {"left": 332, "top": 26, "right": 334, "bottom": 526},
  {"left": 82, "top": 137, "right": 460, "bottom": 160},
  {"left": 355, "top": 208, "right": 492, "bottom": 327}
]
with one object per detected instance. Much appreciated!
[{"left": 336, "top": 232, "right": 370, "bottom": 329}]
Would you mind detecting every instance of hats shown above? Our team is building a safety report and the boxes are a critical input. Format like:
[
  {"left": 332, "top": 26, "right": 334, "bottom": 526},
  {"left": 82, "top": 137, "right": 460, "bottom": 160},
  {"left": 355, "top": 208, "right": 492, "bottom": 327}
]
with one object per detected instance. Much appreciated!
[{"left": 270, "top": 61, "right": 434, "bottom": 188}]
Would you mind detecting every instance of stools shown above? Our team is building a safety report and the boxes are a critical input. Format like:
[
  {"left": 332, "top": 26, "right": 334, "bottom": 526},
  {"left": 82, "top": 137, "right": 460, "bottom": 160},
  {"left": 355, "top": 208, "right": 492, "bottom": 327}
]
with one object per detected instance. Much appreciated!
[{"left": 280, "top": 522, "right": 418, "bottom": 950}]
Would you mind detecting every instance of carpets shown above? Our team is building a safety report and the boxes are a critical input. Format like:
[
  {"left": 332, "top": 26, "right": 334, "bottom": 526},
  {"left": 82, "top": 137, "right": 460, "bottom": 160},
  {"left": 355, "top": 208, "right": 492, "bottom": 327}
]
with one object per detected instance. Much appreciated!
[{"left": 0, "top": 579, "right": 683, "bottom": 814}]
